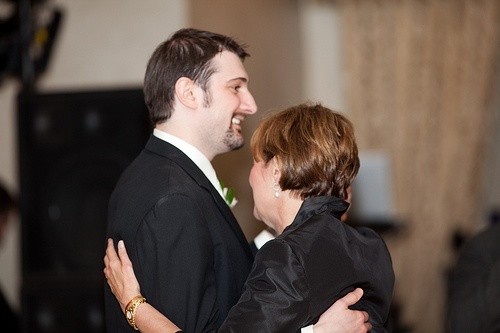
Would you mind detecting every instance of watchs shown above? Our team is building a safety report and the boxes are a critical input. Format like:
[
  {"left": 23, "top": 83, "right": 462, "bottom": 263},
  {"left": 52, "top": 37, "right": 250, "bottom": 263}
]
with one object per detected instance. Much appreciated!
[{"left": 125, "top": 295, "right": 147, "bottom": 331}]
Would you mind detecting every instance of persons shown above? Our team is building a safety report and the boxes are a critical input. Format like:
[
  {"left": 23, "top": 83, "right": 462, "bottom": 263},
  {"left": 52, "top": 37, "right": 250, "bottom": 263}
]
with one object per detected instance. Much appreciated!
[
  {"left": 103, "top": 104, "right": 395, "bottom": 333},
  {"left": 439, "top": 202, "right": 500, "bottom": 332},
  {"left": 104, "top": 29, "right": 373, "bottom": 333}
]
